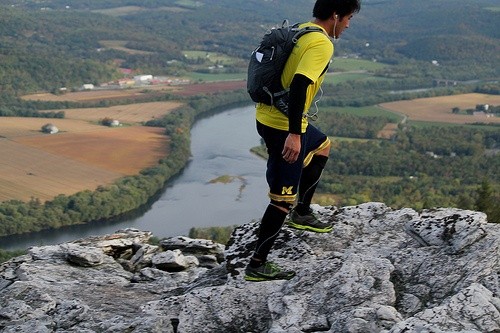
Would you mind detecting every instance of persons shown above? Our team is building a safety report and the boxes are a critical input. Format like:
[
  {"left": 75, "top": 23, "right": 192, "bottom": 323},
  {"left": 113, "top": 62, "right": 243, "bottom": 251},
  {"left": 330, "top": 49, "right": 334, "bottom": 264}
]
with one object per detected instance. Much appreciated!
[{"left": 243, "top": 0, "right": 361, "bottom": 281}]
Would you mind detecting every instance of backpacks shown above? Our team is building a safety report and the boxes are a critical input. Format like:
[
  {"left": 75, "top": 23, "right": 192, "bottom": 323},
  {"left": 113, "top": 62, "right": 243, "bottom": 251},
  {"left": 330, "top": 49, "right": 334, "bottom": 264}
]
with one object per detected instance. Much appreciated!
[{"left": 245, "top": 21, "right": 333, "bottom": 106}]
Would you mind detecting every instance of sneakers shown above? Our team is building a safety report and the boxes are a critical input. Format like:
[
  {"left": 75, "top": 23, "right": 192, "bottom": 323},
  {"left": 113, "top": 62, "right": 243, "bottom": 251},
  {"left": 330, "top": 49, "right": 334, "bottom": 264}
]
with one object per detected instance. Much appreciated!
[
  {"left": 244, "top": 261, "right": 295, "bottom": 281},
  {"left": 289, "top": 211, "right": 333, "bottom": 233}
]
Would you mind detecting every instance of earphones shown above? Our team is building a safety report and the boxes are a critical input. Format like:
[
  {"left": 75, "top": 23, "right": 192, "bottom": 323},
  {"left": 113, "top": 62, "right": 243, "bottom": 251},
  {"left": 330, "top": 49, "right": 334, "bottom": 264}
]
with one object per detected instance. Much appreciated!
[{"left": 335, "top": 15, "right": 338, "bottom": 18}]
